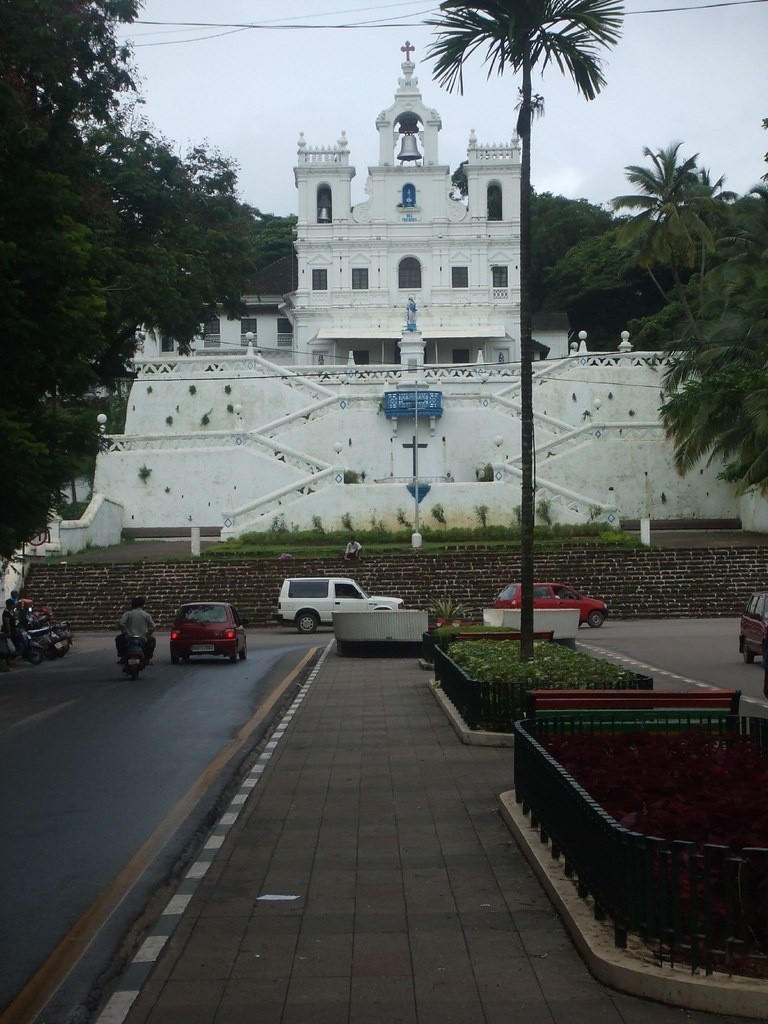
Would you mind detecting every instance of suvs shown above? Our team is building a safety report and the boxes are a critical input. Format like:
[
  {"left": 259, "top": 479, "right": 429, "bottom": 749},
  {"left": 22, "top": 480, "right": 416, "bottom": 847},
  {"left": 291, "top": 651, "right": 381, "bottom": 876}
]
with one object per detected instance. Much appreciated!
[{"left": 272, "top": 577, "right": 405, "bottom": 634}]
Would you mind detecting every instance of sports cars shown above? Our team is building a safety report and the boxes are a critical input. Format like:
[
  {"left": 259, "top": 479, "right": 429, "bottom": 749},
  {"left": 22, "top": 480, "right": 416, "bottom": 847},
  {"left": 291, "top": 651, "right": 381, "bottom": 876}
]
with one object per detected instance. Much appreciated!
[{"left": 121, "top": 633, "right": 150, "bottom": 682}]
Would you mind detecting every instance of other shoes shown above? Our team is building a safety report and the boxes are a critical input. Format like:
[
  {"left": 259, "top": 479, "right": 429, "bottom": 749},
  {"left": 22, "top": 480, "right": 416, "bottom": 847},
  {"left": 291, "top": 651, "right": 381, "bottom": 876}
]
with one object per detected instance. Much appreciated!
[
  {"left": 145, "top": 659, "right": 154, "bottom": 666},
  {"left": 117, "top": 659, "right": 125, "bottom": 664}
]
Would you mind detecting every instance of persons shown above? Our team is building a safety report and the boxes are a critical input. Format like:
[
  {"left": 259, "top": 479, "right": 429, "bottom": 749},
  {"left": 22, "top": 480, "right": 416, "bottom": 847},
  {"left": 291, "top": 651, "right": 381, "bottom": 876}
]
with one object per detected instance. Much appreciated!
[
  {"left": 343, "top": 535, "right": 362, "bottom": 561},
  {"left": 115, "top": 597, "right": 156, "bottom": 665},
  {"left": 2, "top": 591, "right": 19, "bottom": 665},
  {"left": 554, "top": 588, "right": 562, "bottom": 599}
]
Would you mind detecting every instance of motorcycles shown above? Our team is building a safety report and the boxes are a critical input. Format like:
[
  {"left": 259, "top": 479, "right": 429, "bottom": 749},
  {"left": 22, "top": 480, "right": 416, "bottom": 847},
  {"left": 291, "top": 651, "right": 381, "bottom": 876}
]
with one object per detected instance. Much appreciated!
[{"left": 1, "top": 594, "right": 75, "bottom": 664}]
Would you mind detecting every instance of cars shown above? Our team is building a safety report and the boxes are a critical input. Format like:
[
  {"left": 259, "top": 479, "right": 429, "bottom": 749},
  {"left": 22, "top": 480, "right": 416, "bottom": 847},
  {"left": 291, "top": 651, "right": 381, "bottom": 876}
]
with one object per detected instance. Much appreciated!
[
  {"left": 489, "top": 582, "right": 609, "bottom": 629},
  {"left": 739, "top": 590, "right": 768, "bottom": 666},
  {"left": 170, "top": 601, "right": 249, "bottom": 664}
]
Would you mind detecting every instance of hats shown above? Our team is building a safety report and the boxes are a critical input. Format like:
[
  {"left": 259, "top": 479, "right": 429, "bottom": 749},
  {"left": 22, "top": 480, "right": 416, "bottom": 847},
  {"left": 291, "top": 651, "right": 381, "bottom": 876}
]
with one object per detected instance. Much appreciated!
[{"left": 6, "top": 599, "right": 14, "bottom": 605}]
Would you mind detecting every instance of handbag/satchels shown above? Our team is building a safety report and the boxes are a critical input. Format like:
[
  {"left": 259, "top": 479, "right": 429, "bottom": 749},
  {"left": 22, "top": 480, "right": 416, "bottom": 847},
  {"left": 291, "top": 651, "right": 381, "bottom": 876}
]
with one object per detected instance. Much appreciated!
[{"left": 7, "top": 638, "right": 16, "bottom": 653}]
